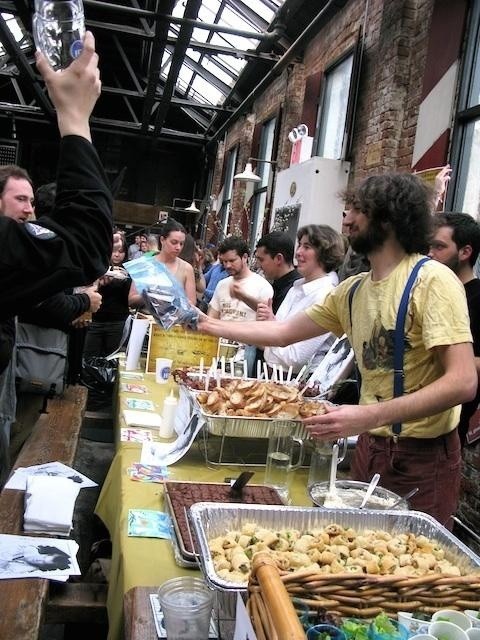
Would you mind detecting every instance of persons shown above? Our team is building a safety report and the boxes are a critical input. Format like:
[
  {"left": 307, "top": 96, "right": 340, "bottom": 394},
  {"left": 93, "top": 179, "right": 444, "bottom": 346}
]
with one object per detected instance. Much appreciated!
[
  {"left": 128, "top": 217, "right": 371, "bottom": 407},
  {"left": 0, "top": 165, "right": 133, "bottom": 489},
  {"left": 177, "top": 172, "right": 479, "bottom": 534},
  {"left": 0, "top": 30, "right": 116, "bottom": 321},
  {"left": 426, "top": 210, "right": 479, "bottom": 450}
]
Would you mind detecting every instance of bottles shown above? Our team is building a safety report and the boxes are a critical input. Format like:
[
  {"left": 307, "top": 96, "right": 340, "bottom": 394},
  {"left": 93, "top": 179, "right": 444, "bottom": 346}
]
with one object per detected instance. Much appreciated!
[
  {"left": 30, "top": 0, "right": 93, "bottom": 74},
  {"left": 155, "top": 388, "right": 179, "bottom": 438}
]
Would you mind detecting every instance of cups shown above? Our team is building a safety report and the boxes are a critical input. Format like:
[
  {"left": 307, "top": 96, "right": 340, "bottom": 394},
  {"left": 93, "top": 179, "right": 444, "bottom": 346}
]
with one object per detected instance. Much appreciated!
[
  {"left": 397, "top": 603, "right": 480, "bottom": 640},
  {"left": 157, "top": 576, "right": 218, "bottom": 640},
  {"left": 308, "top": 424, "right": 347, "bottom": 488},
  {"left": 152, "top": 355, "right": 175, "bottom": 386},
  {"left": 265, "top": 421, "right": 304, "bottom": 489}
]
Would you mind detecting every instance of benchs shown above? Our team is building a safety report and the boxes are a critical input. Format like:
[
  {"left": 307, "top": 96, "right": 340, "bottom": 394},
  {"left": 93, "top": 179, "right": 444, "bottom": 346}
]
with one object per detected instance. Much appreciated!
[{"left": 0, "top": 384, "right": 88, "bottom": 640}]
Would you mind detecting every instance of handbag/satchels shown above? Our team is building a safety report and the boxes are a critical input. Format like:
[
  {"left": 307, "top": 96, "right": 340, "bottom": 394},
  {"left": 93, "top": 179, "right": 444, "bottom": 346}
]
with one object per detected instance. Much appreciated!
[
  {"left": 80, "top": 356, "right": 120, "bottom": 412},
  {"left": 13, "top": 322, "right": 68, "bottom": 399}
]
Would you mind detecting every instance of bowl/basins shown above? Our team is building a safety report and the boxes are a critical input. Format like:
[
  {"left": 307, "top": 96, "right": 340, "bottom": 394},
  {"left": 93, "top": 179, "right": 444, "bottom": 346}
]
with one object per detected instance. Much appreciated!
[{"left": 307, "top": 477, "right": 413, "bottom": 515}]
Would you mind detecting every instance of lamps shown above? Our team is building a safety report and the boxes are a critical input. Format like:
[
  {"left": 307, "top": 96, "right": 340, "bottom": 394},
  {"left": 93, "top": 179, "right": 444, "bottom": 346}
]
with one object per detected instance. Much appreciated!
[
  {"left": 233, "top": 157, "right": 277, "bottom": 183},
  {"left": 185, "top": 199, "right": 209, "bottom": 213}
]
